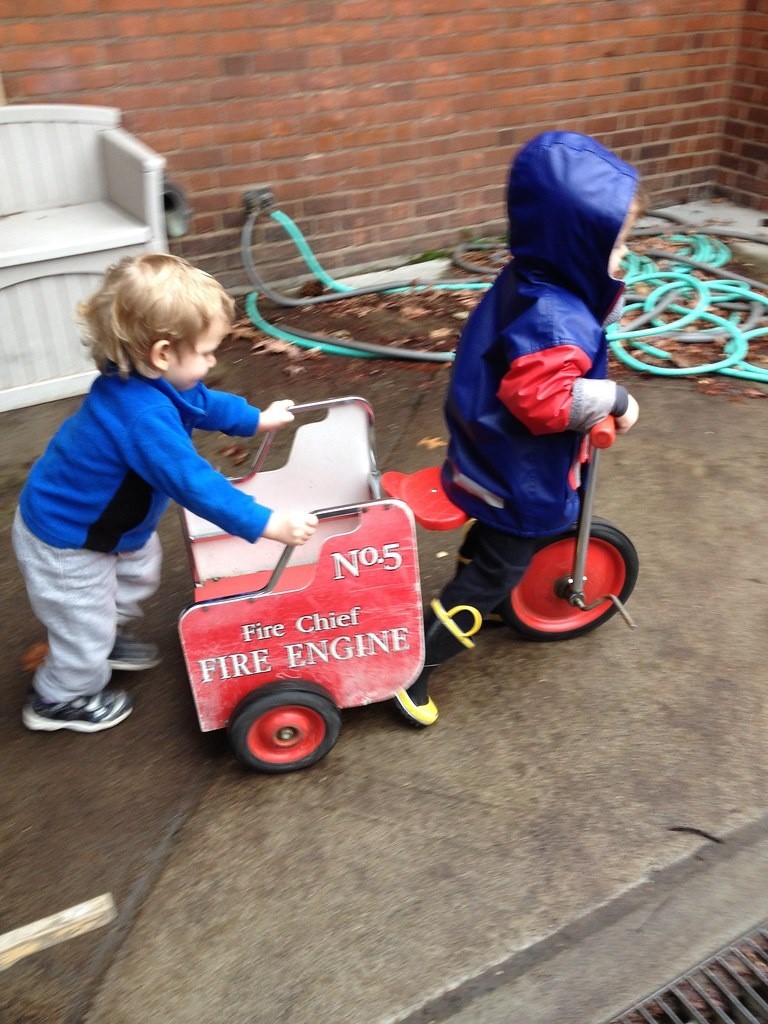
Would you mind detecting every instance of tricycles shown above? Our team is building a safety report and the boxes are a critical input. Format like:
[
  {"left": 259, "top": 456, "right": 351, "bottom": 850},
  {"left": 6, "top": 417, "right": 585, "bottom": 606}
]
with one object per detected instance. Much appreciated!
[{"left": 174, "top": 394, "right": 642, "bottom": 778}]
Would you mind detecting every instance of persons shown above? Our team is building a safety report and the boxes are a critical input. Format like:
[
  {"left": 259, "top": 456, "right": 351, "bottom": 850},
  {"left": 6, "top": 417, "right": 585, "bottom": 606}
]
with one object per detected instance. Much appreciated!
[
  {"left": 10, "top": 253, "right": 319, "bottom": 734},
  {"left": 390, "top": 130, "right": 648, "bottom": 725}
]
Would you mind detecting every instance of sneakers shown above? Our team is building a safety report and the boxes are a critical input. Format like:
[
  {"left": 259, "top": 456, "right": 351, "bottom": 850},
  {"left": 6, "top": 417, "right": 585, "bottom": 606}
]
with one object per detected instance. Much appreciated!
[
  {"left": 21, "top": 683, "right": 134, "bottom": 732},
  {"left": 107, "top": 623, "right": 164, "bottom": 670}
]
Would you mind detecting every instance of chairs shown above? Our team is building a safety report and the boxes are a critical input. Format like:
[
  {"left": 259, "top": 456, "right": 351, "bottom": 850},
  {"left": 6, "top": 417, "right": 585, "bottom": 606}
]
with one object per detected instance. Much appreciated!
[{"left": 0, "top": 102, "right": 168, "bottom": 410}]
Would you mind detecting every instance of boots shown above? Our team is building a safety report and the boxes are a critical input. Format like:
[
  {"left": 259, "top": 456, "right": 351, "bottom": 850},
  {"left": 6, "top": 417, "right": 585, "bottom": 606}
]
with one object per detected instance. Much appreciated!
[
  {"left": 390, "top": 597, "right": 483, "bottom": 728},
  {"left": 454, "top": 517, "right": 504, "bottom": 622}
]
